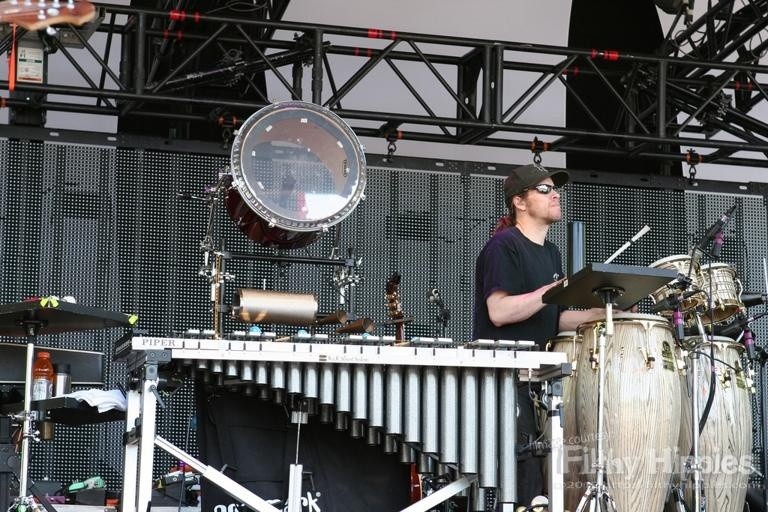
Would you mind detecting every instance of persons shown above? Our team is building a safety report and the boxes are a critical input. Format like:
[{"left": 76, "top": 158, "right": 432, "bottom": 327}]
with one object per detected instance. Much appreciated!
[{"left": 472, "top": 163, "right": 639, "bottom": 507}]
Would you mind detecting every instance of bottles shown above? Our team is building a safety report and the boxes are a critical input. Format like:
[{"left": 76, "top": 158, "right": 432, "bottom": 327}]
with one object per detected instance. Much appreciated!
[{"left": 31, "top": 352, "right": 52, "bottom": 399}]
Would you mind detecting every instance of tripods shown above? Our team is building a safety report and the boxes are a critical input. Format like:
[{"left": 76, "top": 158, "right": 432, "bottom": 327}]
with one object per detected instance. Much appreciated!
[
  {"left": 6, "top": 332, "right": 48, "bottom": 510},
  {"left": 569, "top": 297, "right": 626, "bottom": 511}
]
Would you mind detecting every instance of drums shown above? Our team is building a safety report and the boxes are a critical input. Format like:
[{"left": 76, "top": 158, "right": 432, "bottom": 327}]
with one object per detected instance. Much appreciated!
[
  {"left": 646, "top": 254, "right": 704, "bottom": 315},
  {"left": 690, "top": 265, "right": 743, "bottom": 328},
  {"left": 574, "top": 314, "right": 681, "bottom": 510},
  {"left": 677, "top": 334, "right": 753, "bottom": 511},
  {"left": 541, "top": 331, "right": 583, "bottom": 510},
  {"left": 220, "top": 101, "right": 367, "bottom": 250}
]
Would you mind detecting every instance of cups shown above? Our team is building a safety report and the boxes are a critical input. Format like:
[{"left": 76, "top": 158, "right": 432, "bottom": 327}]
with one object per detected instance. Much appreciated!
[{"left": 52, "top": 363, "right": 72, "bottom": 395}]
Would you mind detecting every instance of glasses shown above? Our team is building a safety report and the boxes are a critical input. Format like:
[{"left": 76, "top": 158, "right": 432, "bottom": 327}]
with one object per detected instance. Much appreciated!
[{"left": 532, "top": 183, "right": 559, "bottom": 193}]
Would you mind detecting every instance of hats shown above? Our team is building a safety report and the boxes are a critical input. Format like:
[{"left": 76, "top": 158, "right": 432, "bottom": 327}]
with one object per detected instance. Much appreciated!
[{"left": 505, "top": 164, "right": 569, "bottom": 203}]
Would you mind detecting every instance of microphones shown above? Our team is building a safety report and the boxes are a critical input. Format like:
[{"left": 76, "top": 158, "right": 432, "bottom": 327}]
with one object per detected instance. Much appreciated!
[
  {"left": 744, "top": 329, "right": 755, "bottom": 363},
  {"left": 720, "top": 314, "right": 762, "bottom": 336},
  {"left": 673, "top": 308, "right": 685, "bottom": 344},
  {"left": 650, "top": 290, "right": 701, "bottom": 313},
  {"left": 689, "top": 205, "right": 738, "bottom": 261}
]
